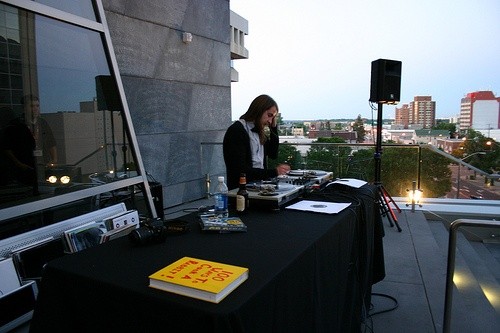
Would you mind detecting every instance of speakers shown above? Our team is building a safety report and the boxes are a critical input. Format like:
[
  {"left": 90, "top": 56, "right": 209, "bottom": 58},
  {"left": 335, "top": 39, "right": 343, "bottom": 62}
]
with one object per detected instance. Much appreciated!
[
  {"left": 95, "top": 74, "right": 121, "bottom": 111},
  {"left": 369, "top": 59, "right": 402, "bottom": 103}
]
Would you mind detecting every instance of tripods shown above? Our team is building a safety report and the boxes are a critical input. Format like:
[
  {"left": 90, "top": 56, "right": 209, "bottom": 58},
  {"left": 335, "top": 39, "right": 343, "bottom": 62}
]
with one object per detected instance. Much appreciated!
[{"left": 374, "top": 103, "right": 404, "bottom": 232}]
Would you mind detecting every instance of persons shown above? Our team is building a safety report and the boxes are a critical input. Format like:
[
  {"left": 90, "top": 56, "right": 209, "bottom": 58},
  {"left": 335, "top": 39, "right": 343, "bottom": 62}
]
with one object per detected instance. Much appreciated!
[
  {"left": 223, "top": 94, "right": 290, "bottom": 191},
  {"left": 0, "top": 94, "right": 58, "bottom": 178}
]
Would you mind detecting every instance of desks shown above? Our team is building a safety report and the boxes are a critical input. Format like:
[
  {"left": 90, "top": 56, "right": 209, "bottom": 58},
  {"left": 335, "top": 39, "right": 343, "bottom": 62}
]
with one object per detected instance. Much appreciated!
[{"left": 27, "top": 182, "right": 386, "bottom": 333}]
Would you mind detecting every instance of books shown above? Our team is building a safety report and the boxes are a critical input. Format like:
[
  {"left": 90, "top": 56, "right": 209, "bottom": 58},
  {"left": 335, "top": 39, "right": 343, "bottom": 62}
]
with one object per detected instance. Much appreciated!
[
  {"left": 148, "top": 257, "right": 248, "bottom": 303},
  {"left": 199, "top": 216, "right": 247, "bottom": 231}
]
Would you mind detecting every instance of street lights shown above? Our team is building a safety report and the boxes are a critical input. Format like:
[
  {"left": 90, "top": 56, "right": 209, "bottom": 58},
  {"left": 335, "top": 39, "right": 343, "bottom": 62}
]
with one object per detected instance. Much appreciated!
[{"left": 456, "top": 151, "right": 487, "bottom": 199}]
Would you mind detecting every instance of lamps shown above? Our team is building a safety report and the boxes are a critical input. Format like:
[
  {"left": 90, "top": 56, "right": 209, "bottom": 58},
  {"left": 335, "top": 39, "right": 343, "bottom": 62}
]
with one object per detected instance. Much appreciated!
[{"left": 406, "top": 188, "right": 424, "bottom": 208}]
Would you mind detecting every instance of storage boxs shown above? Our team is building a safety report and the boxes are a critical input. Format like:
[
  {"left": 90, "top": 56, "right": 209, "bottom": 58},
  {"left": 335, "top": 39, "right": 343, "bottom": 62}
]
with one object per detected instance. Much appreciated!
[{"left": 0, "top": 280, "right": 39, "bottom": 333}]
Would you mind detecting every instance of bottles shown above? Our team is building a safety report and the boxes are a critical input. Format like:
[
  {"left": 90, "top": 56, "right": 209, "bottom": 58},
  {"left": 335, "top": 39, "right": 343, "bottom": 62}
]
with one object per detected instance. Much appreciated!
[
  {"left": 214, "top": 176, "right": 229, "bottom": 217},
  {"left": 236, "top": 173, "right": 249, "bottom": 216}
]
